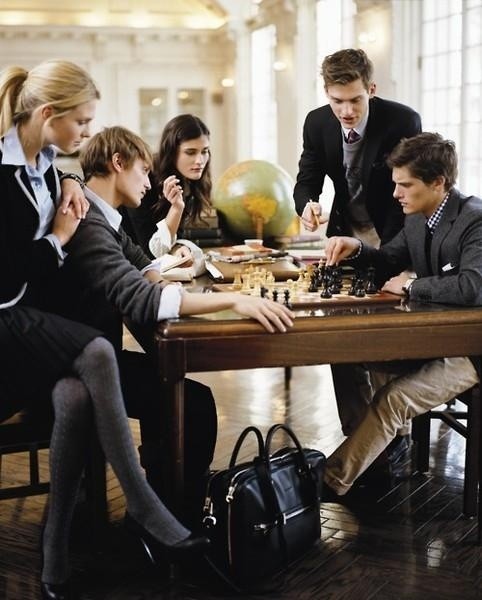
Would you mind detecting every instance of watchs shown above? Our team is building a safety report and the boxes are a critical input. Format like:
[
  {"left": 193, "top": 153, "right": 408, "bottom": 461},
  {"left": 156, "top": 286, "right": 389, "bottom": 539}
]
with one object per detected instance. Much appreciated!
[{"left": 401, "top": 273, "right": 418, "bottom": 295}]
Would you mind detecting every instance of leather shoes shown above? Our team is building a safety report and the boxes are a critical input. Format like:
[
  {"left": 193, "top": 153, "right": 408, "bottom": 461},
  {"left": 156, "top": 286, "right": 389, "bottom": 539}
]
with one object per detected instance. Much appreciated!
[
  {"left": 354, "top": 460, "right": 394, "bottom": 485},
  {"left": 124, "top": 508, "right": 198, "bottom": 558},
  {"left": 41, "top": 580, "right": 68, "bottom": 600}
]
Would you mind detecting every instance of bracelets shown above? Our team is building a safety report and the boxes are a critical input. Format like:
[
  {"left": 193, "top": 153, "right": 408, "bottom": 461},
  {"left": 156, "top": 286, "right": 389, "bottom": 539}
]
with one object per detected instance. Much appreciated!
[{"left": 59, "top": 173, "right": 84, "bottom": 188}]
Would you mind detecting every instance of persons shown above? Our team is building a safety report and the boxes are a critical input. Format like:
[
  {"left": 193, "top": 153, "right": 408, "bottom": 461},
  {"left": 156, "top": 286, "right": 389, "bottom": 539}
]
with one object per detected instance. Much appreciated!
[
  {"left": 324, "top": 132, "right": 481, "bottom": 501},
  {"left": 293, "top": 48, "right": 422, "bottom": 460},
  {"left": 67, "top": 126, "right": 294, "bottom": 581},
  {"left": 2, "top": 59, "right": 216, "bottom": 599},
  {"left": 116, "top": 114, "right": 213, "bottom": 268}
]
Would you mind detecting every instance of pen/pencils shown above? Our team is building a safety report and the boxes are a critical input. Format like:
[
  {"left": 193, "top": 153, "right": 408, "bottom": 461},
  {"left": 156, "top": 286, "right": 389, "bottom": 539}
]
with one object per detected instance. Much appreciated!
[{"left": 309, "top": 200, "right": 321, "bottom": 227}]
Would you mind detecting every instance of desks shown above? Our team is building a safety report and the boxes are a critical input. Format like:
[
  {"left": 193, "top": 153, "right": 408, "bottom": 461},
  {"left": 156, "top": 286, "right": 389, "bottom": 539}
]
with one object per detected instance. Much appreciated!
[{"left": 119, "top": 241, "right": 480, "bottom": 598}]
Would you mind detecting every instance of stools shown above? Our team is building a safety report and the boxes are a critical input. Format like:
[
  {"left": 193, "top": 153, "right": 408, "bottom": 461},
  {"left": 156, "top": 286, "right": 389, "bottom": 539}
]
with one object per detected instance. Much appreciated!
[
  {"left": 2, "top": 403, "right": 116, "bottom": 550},
  {"left": 409, "top": 376, "right": 482, "bottom": 522}
]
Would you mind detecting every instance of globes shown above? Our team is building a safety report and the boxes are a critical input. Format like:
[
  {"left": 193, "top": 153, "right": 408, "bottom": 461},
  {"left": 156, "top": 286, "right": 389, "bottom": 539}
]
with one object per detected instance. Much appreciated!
[{"left": 210, "top": 160, "right": 296, "bottom": 243}]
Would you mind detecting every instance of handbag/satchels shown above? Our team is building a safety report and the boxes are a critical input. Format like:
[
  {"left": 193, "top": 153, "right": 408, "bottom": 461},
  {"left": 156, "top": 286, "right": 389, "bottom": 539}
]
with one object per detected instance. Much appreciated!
[{"left": 202, "top": 424, "right": 327, "bottom": 588}]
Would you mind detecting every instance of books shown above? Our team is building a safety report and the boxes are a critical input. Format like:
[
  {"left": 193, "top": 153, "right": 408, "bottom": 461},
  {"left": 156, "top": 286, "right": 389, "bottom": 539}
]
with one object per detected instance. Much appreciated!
[{"left": 218, "top": 243, "right": 272, "bottom": 257}]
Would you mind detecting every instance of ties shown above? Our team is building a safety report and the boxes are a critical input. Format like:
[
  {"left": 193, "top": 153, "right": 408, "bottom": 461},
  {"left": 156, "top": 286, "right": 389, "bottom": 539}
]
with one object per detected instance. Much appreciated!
[{"left": 348, "top": 130, "right": 355, "bottom": 144}]
